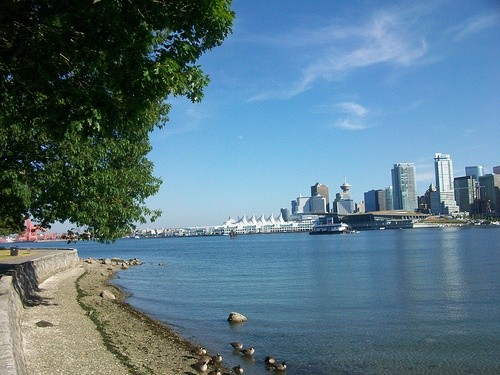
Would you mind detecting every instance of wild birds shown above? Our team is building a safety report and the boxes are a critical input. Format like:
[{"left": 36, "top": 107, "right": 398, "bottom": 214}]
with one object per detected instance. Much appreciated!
[
  {"left": 192, "top": 362, "right": 208, "bottom": 372},
  {"left": 265, "top": 356, "right": 275, "bottom": 363},
  {"left": 227, "top": 312, "right": 248, "bottom": 322},
  {"left": 207, "top": 368, "right": 223, "bottom": 375},
  {"left": 233, "top": 365, "right": 244, "bottom": 375},
  {"left": 215, "top": 353, "right": 224, "bottom": 367},
  {"left": 199, "top": 353, "right": 213, "bottom": 366},
  {"left": 229, "top": 342, "right": 245, "bottom": 349},
  {"left": 192, "top": 347, "right": 208, "bottom": 355},
  {"left": 240, "top": 347, "right": 254, "bottom": 356},
  {"left": 272, "top": 361, "right": 287, "bottom": 370}
]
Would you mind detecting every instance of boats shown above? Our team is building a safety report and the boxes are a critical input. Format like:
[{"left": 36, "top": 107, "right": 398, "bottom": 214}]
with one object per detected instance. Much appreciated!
[{"left": 308, "top": 223, "right": 348, "bottom": 235}]
